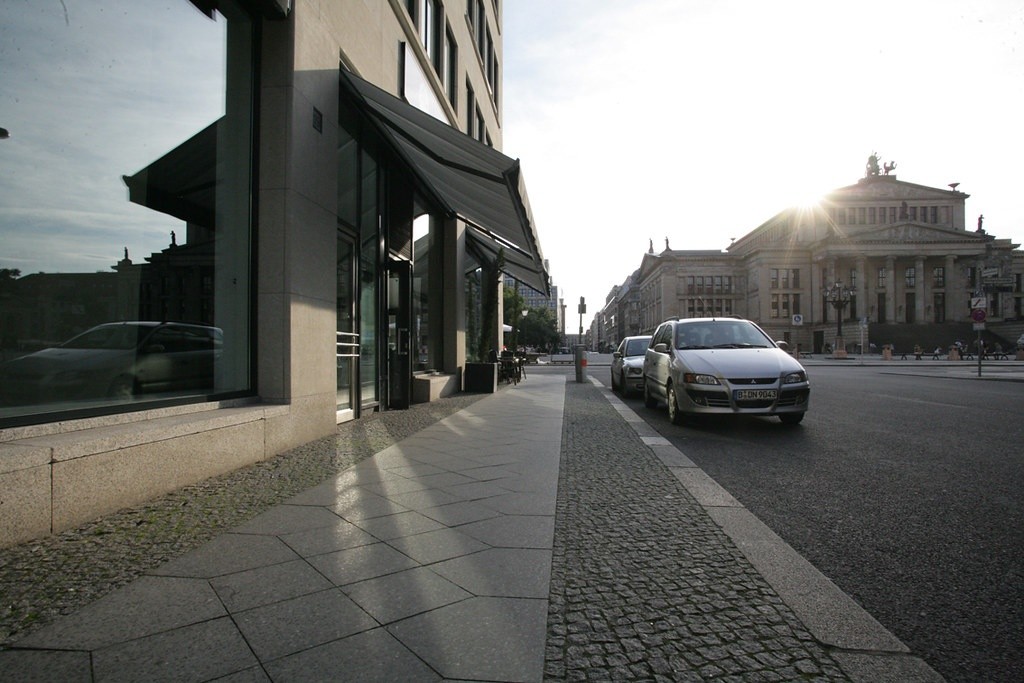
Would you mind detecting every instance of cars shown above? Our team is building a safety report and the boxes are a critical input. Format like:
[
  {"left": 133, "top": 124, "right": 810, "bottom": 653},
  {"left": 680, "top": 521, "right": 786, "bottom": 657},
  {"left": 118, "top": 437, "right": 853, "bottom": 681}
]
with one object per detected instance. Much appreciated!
[{"left": 610, "top": 336, "right": 651, "bottom": 398}]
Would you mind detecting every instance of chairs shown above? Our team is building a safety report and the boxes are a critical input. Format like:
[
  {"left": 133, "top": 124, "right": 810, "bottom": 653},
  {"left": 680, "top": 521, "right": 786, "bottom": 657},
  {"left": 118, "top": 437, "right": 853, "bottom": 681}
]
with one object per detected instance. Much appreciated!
[{"left": 488, "top": 348, "right": 527, "bottom": 385}]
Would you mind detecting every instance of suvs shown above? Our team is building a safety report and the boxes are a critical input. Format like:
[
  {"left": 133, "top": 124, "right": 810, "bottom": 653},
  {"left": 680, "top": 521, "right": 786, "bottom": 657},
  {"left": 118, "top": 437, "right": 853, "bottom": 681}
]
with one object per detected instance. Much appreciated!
[
  {"left": 643, "top": 314, "right": 810, "bottom": 425},
  {"left": 2, "top": 319, "right": 224, "bottom": 407}
]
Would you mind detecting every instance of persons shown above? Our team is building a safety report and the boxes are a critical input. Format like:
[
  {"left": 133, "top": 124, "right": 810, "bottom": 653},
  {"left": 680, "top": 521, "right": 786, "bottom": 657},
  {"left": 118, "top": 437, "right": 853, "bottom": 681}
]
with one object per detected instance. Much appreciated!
[
  {"left": 933, "top": 344, "right": 942, "bottom": 360},
  {"left": 993, "top": 342, "right": 1002, "bottom": 360},
  {"left": 954, "top": 339, "right": 968, "bottom": 353},
  {"left": 824, "top": 341, "right": 832, "bottom": 354},
  {"left": 1016, "top": 334, "right": 1024, "bottom": 345},
  {"left": 973, "top": 338, "right": 989, "bottom": 360}
]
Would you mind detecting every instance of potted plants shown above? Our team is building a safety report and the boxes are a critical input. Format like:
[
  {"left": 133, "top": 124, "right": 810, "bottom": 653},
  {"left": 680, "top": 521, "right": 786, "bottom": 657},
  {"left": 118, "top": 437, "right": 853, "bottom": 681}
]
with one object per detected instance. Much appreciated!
[{"left": 464, "top": 245, "right": 505, "bottom": 394}]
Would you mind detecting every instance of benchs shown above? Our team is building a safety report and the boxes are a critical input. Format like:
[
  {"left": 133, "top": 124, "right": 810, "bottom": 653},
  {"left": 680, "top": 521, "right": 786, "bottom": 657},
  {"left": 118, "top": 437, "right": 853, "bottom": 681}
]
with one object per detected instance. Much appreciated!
[{"left": 891, "top": 352, "right": 1009, "bottom": 361}]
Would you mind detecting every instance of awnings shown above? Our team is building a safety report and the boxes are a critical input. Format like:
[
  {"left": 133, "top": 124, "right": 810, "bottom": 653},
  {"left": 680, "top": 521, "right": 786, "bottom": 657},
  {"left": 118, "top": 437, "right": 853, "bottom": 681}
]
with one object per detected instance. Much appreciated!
[{"left": 342, "top": 68, "right": 551, "bottom": 296}]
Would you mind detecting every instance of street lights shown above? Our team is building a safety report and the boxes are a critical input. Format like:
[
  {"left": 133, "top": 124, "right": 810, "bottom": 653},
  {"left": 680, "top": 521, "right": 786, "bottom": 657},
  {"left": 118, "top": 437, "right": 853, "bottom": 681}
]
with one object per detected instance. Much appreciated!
[
  {"left": 522, "top": 306, "right": 530, "bottom": 353},
  {"left": 823, "top": 279, "right": 855, "bottom": 337}
]
